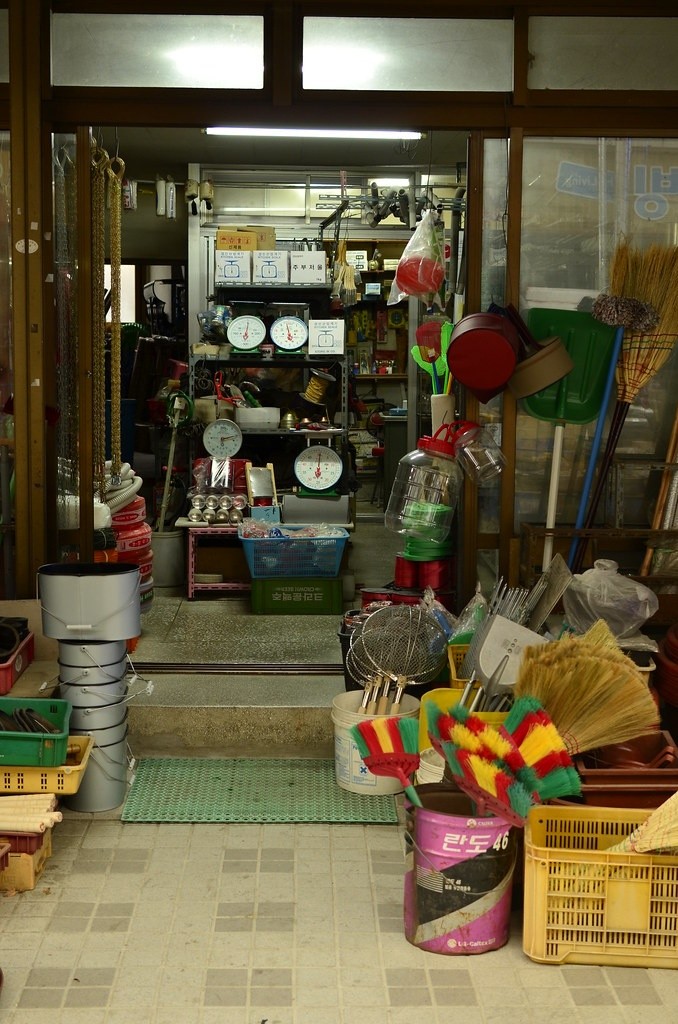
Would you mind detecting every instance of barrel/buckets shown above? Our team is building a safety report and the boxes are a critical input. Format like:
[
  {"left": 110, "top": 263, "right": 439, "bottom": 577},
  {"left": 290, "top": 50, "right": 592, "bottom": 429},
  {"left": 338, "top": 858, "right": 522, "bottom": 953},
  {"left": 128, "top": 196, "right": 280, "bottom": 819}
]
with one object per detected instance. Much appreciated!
[
  {"left": 331, "top": 690, "right": 420, "bottom": 795},
  {"left": 36, "top": 561, "right": 154, "bottom": 812},
  {"left": 384, "top": 424, "right": 464, "bottom": 543},
  {"left": 403, "top": 783, "right": 516, "bottom": 955},
  {"left": 416, "top": 747, "right": 445, "bottom": 785},
  {"left": 448, "top": 420, "right": 507, "bottom": 483}
]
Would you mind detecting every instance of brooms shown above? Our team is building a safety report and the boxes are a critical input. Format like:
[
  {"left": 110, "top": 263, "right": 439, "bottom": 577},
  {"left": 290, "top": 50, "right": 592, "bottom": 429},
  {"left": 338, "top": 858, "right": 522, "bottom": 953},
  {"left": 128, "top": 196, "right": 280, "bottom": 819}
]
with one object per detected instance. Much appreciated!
[
  {"left": 349, "top": 615, "right": 663, "bottom": 830},
  {"left": 569, "top": 232, "right": 678, "bottom": 575}
]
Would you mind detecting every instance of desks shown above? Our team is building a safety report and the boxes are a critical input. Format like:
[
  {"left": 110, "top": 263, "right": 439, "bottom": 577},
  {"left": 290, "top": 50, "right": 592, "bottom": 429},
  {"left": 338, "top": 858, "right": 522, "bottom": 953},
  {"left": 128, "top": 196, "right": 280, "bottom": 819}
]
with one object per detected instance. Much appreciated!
[
  {"left": 188, "top": 528, "right": 252, "bottom": 599},
  {"left": 379, "top": 412, "right": 408, "bottom": 513}
]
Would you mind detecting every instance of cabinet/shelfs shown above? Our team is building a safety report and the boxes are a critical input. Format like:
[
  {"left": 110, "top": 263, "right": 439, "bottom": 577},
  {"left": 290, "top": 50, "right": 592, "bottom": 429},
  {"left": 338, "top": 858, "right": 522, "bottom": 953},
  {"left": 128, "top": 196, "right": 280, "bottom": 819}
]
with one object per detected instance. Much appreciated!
[{"left": 205, "top": 234, "right": 460, "bottom": 524}]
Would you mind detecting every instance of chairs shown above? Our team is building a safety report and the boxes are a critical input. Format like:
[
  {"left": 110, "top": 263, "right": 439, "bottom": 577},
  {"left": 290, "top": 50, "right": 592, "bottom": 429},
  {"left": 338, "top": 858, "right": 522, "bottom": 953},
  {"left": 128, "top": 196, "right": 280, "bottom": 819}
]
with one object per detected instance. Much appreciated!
[{"left": 370, "top": 411, "right": 396, "bottom": 514}]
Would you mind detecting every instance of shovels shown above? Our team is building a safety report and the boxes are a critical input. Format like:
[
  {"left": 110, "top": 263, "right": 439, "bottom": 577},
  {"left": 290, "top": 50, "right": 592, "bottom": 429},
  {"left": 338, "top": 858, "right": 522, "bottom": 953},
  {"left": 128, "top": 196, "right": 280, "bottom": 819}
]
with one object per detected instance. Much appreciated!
[
  {"left": 476, "top": 613, "right": 549, "bottom": 712},
  {"left": 521, "top": 308, "right": 621, "bottom": 573}
]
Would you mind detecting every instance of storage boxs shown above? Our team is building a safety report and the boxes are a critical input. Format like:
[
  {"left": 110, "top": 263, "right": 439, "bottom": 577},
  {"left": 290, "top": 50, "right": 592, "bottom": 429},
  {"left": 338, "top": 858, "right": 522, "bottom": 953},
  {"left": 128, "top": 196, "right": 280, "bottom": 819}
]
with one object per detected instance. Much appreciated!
[
  {"left": 335, "top": 429, "right": 378, "bottom": 471},
  {"left": 215, "top": 222, "right": 328, "bottom": 286},
  {"left": 0, "top": 630, "right": 35, "bottom": 696},
  {"left": 0, "top": 734, "right": 95, "bottom": 795},
  {"left": 336, "top": 620, "right": 487, "bottom": 700},
  {"left": 195, "top": 394, "right": 281, "bottom": 430},
  {"left": 309, "top": 320, "right": 347, "bottom": 354},
  {"left": 238, "top": 522, "right": 351, "bottom": 616},
  {"left": 522, "top": 654, "right": 678, "bottom": 972},
  {"left": 0, "top": 697, "right": 73, "bottom": 767},
  {"left": 0, "top": 824, "right": 52, "bottom": 891}
]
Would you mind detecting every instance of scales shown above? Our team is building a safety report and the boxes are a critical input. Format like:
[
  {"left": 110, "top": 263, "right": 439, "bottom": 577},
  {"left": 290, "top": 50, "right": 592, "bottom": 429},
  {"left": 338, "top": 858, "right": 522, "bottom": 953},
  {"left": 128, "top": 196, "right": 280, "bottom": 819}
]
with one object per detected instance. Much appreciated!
[
  {"left": 226, "top": 300, "right": 266, "bottom": 357},
  {"left": 293, "top": 429, "right": 346, "bottom": 497},
  {"left": 267, "top": 302, "right": 309, "bottom": 358}
]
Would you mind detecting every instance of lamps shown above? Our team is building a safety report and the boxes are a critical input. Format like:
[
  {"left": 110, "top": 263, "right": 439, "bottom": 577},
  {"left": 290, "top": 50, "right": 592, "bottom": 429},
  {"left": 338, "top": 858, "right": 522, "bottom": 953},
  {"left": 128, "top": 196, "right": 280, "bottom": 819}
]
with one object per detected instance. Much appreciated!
[{"left": 200, "top": 127, "right": 428, "bottom": 140}]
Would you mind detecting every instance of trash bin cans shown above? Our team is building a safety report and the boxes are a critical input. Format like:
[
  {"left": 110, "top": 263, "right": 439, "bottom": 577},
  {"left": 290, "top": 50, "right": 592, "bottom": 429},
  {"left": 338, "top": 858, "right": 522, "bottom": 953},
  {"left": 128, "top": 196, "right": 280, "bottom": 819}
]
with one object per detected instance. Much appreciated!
[{"left": 380, "top": 410, "right": 408, "bottom": 513}]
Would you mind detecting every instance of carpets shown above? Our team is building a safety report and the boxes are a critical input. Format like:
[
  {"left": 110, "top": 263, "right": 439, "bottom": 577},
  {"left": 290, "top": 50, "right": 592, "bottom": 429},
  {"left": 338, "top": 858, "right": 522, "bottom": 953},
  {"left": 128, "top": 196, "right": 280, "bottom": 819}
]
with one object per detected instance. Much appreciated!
[{"left": 119, "top": 757, "right": 399, "bottom": 826}]
